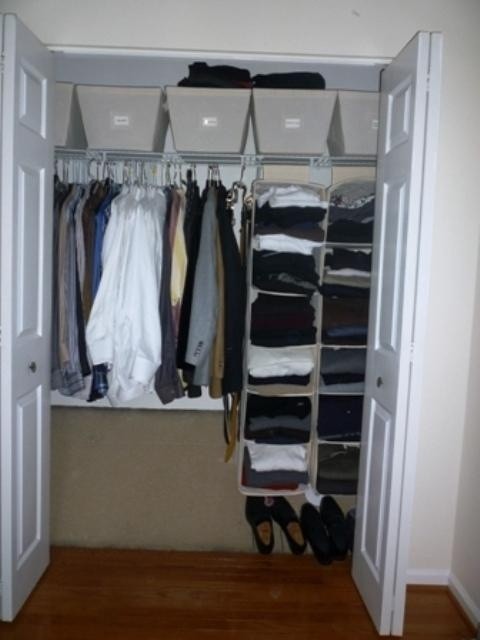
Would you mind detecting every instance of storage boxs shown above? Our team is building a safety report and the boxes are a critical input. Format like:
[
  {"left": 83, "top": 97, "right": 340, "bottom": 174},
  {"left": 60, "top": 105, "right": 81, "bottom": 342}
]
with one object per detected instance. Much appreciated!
[
  {"left": 160, "top": 83, "right": 254, "bottom": 155},
  {"left": 74, "top": 82, "right": 170, "bottom": 153},
  {"left": 48, "top": 81, "right": 82, "bottom": 145},
  {"left": 251, "top": 88, "right": 337, "bottom": 156},
  {"left": 329, "top": 92, "right": 383, "bottom": 157}
]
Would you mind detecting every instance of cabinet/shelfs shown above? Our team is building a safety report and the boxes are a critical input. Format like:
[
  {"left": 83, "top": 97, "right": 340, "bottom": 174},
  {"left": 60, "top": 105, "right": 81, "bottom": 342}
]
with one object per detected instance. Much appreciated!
[{"left": 2, "top": 11, "right": 447, "bottom": 640}]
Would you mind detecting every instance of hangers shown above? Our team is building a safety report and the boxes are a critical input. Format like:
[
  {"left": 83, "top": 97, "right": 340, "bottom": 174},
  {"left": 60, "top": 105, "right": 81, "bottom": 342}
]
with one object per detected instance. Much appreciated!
[{"left": 52, "top": 149, "right": 229, "bottom": 191}]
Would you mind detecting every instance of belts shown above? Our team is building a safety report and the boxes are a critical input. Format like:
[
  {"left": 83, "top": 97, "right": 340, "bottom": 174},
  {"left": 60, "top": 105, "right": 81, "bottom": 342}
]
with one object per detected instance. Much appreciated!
[{"left": 223, "top": 187, "right": 253, "bottom": 464}]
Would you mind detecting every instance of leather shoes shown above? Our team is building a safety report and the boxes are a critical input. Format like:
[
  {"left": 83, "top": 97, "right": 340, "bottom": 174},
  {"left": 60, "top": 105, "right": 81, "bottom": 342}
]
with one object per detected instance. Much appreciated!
[
  {"left": 269, "top": 496, "right": 308, "bottom": 555},
  {"left": 300, "top": 503, "right": 333, "bottom": 565},
  {"left": 321, "top": 496, "right": 348, "bottom": 561},
  {"left": 246, "top": 495, "right": 275, "bottom": 555}
]
